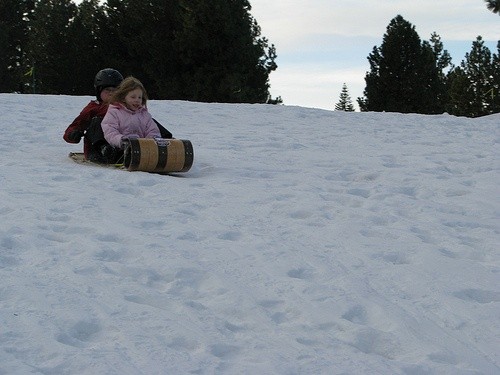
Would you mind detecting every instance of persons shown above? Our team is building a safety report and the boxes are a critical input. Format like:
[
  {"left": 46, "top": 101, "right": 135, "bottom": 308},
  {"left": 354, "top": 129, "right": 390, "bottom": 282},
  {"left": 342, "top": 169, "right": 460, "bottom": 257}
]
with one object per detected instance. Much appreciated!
[
  {"left": 101, "top": 75, "right": 162, "bottom": 164},
  {"left": 63, "top": 68, "right": 177, "bottom": 164}
]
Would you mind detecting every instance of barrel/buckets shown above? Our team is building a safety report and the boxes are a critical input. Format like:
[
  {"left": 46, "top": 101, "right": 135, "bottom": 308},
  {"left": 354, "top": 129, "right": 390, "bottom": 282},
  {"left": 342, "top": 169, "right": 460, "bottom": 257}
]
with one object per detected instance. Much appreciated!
[{"left": 124, "top": 138, "right": 194, "bottom": 171}]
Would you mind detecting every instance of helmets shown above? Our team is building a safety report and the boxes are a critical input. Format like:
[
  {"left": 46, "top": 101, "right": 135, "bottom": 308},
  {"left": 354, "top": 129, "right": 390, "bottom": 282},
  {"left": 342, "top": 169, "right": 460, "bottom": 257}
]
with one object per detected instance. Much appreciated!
[{"left": 94, "top": 68, "right": 124, "bottom": 89}]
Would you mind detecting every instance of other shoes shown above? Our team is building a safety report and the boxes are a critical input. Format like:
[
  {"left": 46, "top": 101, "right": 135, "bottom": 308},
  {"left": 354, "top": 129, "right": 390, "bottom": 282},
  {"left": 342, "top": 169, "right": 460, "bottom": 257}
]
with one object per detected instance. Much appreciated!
[{"left": 100, "top": 143, "right": 113, "bottom": 163}]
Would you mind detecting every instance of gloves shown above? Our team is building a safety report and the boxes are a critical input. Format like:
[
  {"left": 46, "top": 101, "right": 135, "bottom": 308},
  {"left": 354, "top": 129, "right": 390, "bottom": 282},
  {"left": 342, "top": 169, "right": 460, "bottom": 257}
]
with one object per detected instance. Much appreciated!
[{"left": 70, "top": 131, "right": 84, "bottom": 143}]
